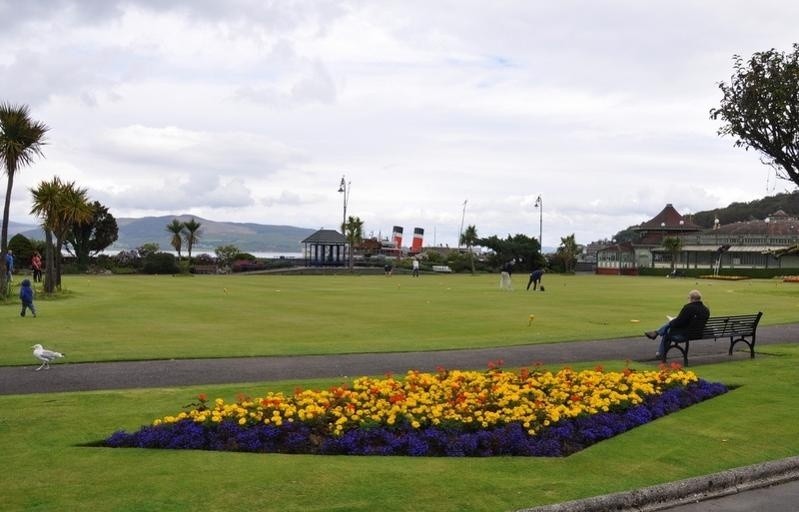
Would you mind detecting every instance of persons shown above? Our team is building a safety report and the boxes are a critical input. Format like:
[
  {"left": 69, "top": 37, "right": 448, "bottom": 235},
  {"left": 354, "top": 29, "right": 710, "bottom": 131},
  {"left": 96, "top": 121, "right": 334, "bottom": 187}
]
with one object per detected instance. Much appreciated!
[
  {"left": 643, "top": 289, "right": 710, "bottom": 358},
  {"left": 413, "top": 257, "right": 420, "bottom": 276},
  {"left": 527, "top": 268, "right": 545, "bottom": 292},
  {"left": 19, "top": 278, "right": 37, "bottom": 317},
  {"left": 31, "top": 250, "right": 43, "bottom": 282},
  {"left": 499, "top": 257, "right": 516, "bottom": 290},
  {"left": 6, "top": 249, "right": 14, "bottom": 283},
  {"left": 384, "top": 264, "right": 393, "bottom": 275},
  {"left": 36, "top": 255, "right": 43, "bottom": 282}
]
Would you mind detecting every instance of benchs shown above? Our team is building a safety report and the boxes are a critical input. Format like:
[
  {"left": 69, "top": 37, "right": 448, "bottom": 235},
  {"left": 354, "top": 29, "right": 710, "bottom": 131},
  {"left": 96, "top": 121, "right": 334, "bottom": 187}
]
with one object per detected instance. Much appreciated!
[{"left": 657, "top": 310, "right": 766, "bottom": 367}]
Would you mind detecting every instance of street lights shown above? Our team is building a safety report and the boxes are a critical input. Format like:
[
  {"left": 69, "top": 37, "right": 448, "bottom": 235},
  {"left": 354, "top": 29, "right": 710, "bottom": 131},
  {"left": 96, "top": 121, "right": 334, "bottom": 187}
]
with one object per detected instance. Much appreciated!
[
  {"left": 337, "top": 175, "right": 351, "bottom": 236},
  {"left": 764, "top": 217, "right": 770, "bottom": 246},
  {"left": 660, "top": 221, "right": 666, "bottom": 244},
  {"left": 534, "top": 195, "right": 543, "bottom": 255},
  {"left": 679, "top": 219, "right": 684, "bottom": 245},
  {"left": 715, "top": 218, "right": 719, "bottom": 246}
]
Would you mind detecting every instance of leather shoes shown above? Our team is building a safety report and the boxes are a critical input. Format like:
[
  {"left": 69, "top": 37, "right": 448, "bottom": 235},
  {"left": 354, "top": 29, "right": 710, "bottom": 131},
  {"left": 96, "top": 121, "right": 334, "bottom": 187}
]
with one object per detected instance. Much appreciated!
[
  {"left": 645, "top": 331, "right": 658, "bottom": 340},
  {"left": 655, "top": 351, "right": 662, "bottom": 360}
]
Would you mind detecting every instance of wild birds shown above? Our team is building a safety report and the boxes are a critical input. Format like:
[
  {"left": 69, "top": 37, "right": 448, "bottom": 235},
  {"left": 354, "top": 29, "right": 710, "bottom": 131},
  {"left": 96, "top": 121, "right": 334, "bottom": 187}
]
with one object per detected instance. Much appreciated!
[{"left": 31, "top": 344, "right": 64, "bottom": 371}]
